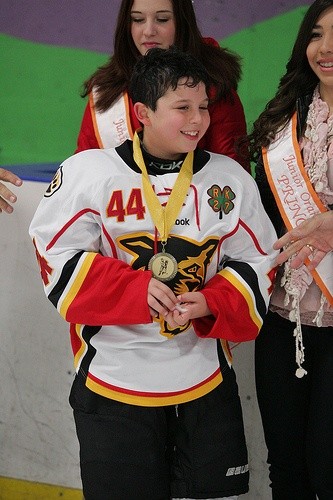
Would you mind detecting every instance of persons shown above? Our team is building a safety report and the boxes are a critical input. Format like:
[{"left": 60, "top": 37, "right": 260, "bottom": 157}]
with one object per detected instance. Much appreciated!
[
  {"left": 235, "top": 0, "right": 333, "bottom": 500},
  {"left": 29, "top": 48, "right": 280, "bottom": 500},
  {"left": 74, "top": 0, "right": 252, "bottom": 175},
  {"left": 0, "top": 168, "right": 23, "bottom": 214}
]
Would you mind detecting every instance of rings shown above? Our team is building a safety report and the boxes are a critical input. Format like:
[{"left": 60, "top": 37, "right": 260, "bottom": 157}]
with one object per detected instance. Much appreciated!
[{"left": 305, "top": 242, "right": 314, "bottom": 253}]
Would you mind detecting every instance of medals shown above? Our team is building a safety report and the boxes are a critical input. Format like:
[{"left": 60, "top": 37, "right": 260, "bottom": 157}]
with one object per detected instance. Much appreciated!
[{"left": 148, "top": 240, "right": 178, "bottom": 282}]
